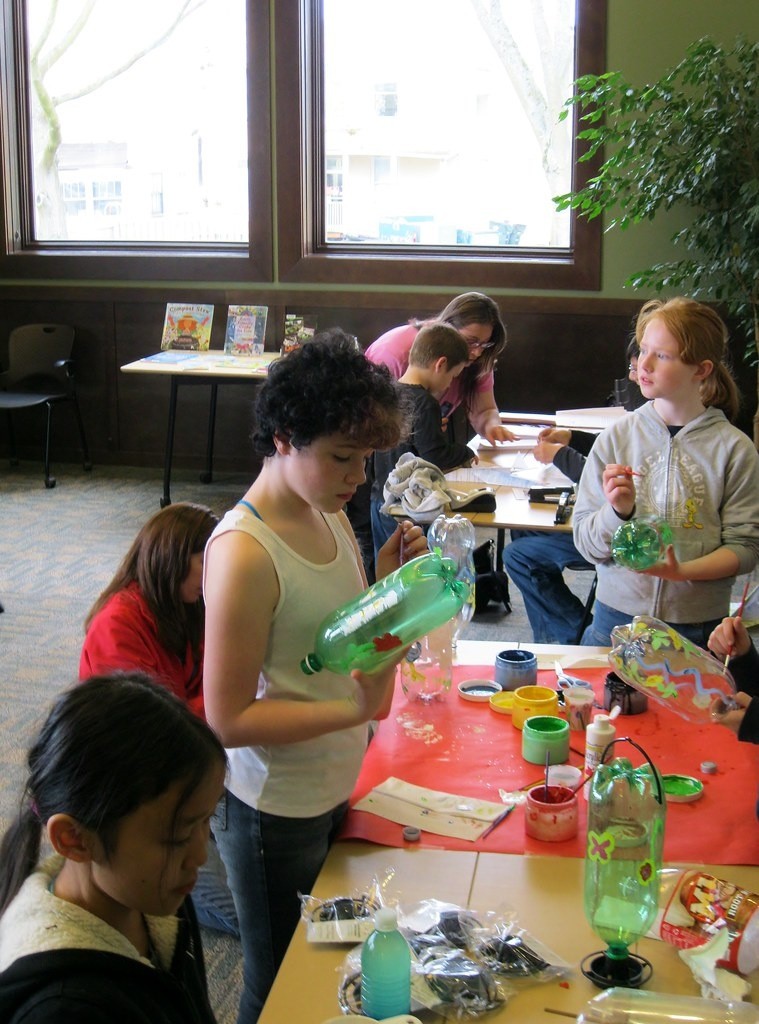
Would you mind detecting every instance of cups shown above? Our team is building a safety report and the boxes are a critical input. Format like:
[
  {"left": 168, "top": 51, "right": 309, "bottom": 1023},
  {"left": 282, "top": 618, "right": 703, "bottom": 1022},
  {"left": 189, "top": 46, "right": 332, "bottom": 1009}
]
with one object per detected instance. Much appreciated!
[
  {"left": 520, "top": 715, "right": 570, "bottom": 765},
  {"left": 543, "top": 765, "right": 582, "bottom": 796},
  {"left": 525, "top": 783, "right": 582, "bottom": 843},
  {"left": 511, "top": 685, "right": 560, "bottom": 730},
  {"left": 494, "top": 649, "right": 537, "bottom": 691}
]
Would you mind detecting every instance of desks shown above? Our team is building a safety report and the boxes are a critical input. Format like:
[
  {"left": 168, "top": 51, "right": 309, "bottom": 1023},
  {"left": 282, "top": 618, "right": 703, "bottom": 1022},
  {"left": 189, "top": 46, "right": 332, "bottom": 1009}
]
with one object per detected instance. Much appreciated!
[
  {"left": 389, "top": 406, "right": 634, "bottom": 645},
  {"left": 254, "top": 641, "right": 758, "bottom": 1024},
  {"left": 120, "top": 349, "right": 292, "bottom": 509}
]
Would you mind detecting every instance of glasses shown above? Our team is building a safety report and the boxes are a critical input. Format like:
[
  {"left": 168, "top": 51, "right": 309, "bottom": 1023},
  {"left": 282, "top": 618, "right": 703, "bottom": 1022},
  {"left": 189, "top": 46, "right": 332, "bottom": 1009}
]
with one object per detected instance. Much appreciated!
[
  {"left": 466, "top": 340, "right": 495, "bottom": 349},
  {"left": 629, "top": 364, "right": 637, "bottom": 371}
]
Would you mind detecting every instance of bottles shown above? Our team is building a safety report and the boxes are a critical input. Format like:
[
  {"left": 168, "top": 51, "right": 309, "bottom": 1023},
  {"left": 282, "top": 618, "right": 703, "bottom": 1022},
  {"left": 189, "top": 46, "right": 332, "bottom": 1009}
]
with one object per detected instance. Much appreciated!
[
  {"left": 576, "top": 985, "right": 759, "bottom": 1024},
  {"left": 401, "top": 619, "right": 454, "bottom": 702},
  {"left": 613, "top": 868, "right": 759, "bottom": 976},
  {"left": 582, "top": 756, "right": 666, "bottom": 959},
  {"left": 604, "top": 614, "right": 744, "bottom": 725},
  {"left": 563, "top": 686, "right": 595, "bottom": 732},
  {"left": 299, "top": 552, "right": 475, "bottom": 675},
  {"left": 360, "top": 908, "right": 411, "bottom": 1021},
  {"left": 610, "top": 518, "right": 671, "bottom": 569},
  {"left": 584, "top": 706, "right": 622, "bottom": 770},
  {"left": 425, "top": 512, "right": 475, "bottom": 657}
]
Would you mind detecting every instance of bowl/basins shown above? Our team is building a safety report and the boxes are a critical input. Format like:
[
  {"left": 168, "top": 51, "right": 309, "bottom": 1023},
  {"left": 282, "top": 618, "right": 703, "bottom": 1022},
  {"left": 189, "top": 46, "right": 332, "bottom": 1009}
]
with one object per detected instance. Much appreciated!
[{"left": 602, "top": 670, "right": 650, "bottom": 715}]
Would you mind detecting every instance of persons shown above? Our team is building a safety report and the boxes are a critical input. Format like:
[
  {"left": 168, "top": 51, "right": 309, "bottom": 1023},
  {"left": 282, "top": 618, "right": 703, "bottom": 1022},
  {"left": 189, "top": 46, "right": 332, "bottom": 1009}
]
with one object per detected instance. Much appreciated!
[
  {"left": 75, "top": 501, "right": 242, "bottom": 936},
  {"left": 347, "top": 293, "right": 518, "bottom": 586},
  {"left": 502, "top": 294, "right": 759, "bottom": 743},
  {"left": 203, "top": 328, "right": 433, "bottom": 1024},
  {"left": 0, "top": 673, "right": 230, "bottom": 1024}
]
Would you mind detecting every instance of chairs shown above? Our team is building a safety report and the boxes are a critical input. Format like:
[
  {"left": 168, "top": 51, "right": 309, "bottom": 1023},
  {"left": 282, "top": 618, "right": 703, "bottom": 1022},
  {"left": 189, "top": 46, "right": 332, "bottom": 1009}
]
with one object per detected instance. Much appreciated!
[{"left": 0, "top": 324, "right": 93, "bottom": 488}]
